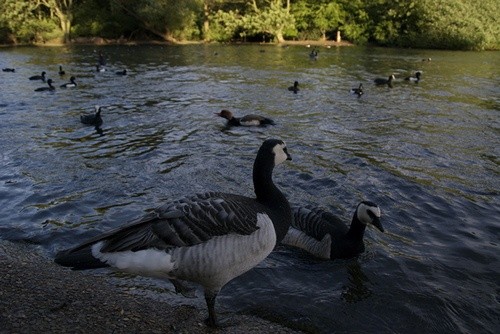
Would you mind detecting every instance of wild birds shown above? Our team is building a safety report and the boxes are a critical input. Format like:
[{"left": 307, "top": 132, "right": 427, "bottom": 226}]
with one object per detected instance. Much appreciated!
[
  {"left": 60, "top": 76, "right": 78, "bottom": 87},
  {"left": 33, "top": 78, "right": 56, "bottom": 92},
  {"left": 79, "top": 105, "right": 104, "bottom": 127},
  {"left": 28, "top": 71, "right": 48, "bottom": 80},
  {"left": 56, "top": 63, "right": 65, "bottom": 75},
  {"left": 52, "top": 138, "right": 296, "bottom": 331},
  {"left": 282, "top": 43, "right": 331, "bottom": 57},
  {"left": 404, "top": 72, "right": 425, "bottom": 82},
  {"left": 93, "top": 62, "right": 106, "bottom": 73},
  {"left": 213, "top": 108, "right": 276, "bottom": 133},
  {"left": 285, "top": 200, "right": 387, "bottom": 262},
  {"left": 288, "top": 81, "right": 300, "bottom": 92},
  {"left": 115, "top": 64, "right": 171, "bottom": 75},
  {"left": 420, "top": 55, "right": 433, "bottom": 63},
  {"left": 351, "top": 82, "right": 365, "bottom": 95},
  {"left": 3, "top": 66, "right": 16, "bottom": 73},
  {"left": 374, "top": 74, "right": 396, "bottom": 88}
]
